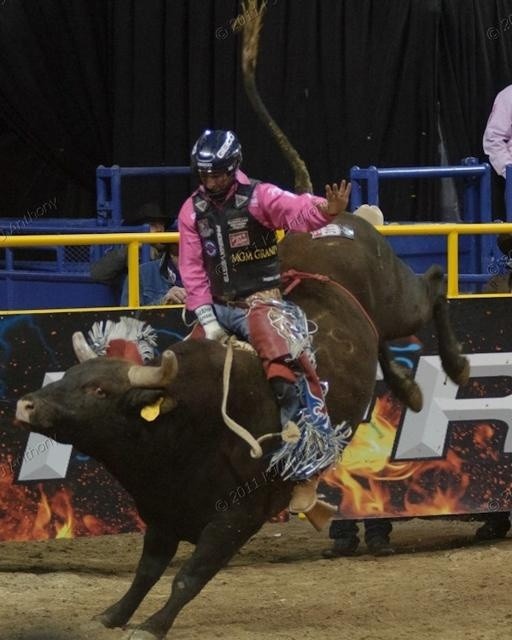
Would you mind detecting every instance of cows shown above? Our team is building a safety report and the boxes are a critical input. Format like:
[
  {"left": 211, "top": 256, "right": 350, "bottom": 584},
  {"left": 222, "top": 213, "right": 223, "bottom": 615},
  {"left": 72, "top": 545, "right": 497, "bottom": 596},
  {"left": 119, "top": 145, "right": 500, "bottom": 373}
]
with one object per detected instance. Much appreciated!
[{"left": 15, "top": 0, "right": 471, "bottom": 639}]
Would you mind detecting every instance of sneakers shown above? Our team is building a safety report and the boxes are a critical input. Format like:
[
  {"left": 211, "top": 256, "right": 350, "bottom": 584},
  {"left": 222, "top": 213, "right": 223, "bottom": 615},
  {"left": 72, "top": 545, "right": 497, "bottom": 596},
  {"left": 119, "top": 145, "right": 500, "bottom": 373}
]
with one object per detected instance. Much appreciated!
[{"left": 321, "top": 519, "right": 511, "bottom": 558}]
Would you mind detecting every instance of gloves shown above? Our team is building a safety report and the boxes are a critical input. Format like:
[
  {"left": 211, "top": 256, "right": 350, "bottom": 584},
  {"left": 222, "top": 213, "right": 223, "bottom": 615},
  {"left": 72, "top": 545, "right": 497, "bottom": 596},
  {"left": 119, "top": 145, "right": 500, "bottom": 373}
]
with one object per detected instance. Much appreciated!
[
  {"left": 194, "top": 302, "right": 229, "bottom": 343},
  {"left": 161, "top": 285, "right": 190, "bottom": 306}
]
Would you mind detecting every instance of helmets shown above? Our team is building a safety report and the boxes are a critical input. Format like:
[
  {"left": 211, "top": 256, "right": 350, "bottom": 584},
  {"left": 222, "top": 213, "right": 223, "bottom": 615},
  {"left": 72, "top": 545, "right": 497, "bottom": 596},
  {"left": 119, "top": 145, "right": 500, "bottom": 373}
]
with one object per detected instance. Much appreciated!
[{"left": 189, "top": 129, "right": 243, "bottom": 187}]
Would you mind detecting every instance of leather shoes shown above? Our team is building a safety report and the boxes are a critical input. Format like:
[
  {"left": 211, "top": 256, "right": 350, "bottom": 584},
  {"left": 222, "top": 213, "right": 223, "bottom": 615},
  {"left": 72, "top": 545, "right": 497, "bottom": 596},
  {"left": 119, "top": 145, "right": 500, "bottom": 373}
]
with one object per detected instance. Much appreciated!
[{"left": 288, "top": 470, "right": 323, "bottom": 515}]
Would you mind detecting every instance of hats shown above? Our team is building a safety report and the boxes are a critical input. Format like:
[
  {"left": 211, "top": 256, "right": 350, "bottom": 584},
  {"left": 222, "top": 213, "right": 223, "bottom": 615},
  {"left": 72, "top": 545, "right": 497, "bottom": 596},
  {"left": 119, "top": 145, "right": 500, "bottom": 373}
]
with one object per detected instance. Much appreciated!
[
  {"left": 348, "top": 203, "right": 400, "bottom": 226},
  {"left": 119, "top": 200, "right": 174, "bottom": 226}
]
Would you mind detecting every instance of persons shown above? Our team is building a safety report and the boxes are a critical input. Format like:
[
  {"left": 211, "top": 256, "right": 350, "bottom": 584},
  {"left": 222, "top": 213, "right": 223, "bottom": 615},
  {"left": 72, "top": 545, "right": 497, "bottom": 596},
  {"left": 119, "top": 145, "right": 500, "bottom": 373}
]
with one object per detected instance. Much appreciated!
[
  {"left": 121, "top": 218, "right": 187, "bottom": 307},
  {"left": 474, "top": 233, "right": 512, "bottom": 540},
  {"left": 178, "top": 130, "right": 352, "bottom": 477},
  {"left": 482, "top": 84, "right": 512, "bottom": 180},
  {"left": 88, "top": 203, "right": 172, "bottom": 306},
  {"left": 321, "top": 519, "right": 395, "bottom": 558}
]
